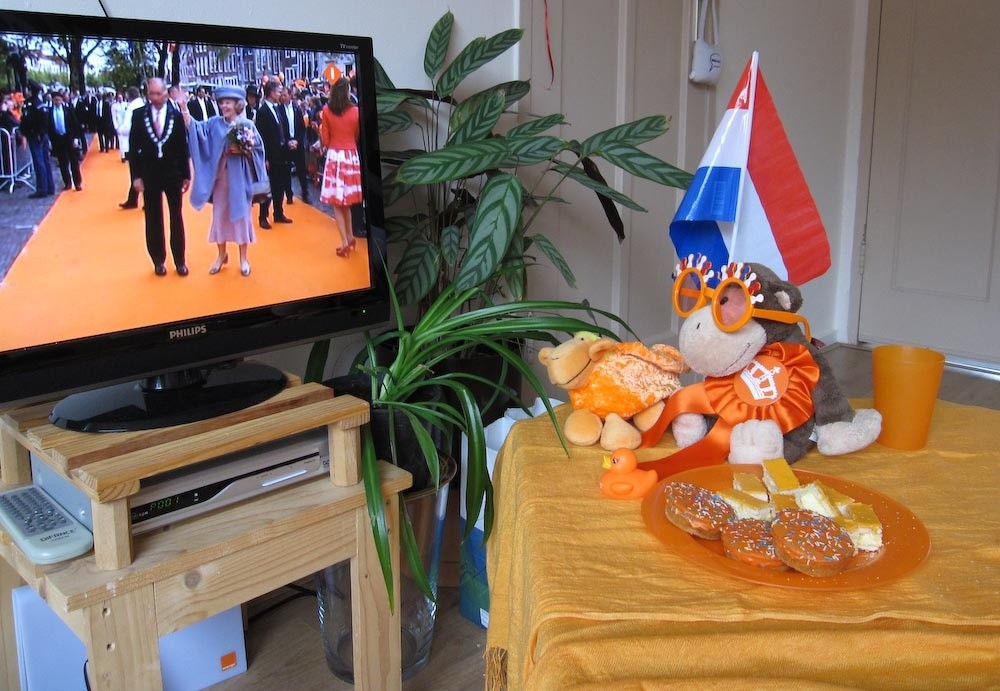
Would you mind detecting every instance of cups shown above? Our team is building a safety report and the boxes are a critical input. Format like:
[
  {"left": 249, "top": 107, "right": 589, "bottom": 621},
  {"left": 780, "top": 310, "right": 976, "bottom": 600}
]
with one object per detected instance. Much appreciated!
[{"left": 870, "top": 344, "right": 946, "bottom": 452}]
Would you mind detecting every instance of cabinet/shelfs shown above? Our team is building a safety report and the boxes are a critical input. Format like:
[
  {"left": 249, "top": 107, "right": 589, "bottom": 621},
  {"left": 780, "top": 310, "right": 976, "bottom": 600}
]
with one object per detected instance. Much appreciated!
[{"left": 0, "top": 366, "right": 415, "bottom": 691}]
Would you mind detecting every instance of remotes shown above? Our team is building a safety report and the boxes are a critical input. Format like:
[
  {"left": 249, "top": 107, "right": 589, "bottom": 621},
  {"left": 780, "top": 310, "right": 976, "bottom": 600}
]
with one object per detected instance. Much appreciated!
[{"left": 0, "top": 484, "right": 94, "bottom": 564}]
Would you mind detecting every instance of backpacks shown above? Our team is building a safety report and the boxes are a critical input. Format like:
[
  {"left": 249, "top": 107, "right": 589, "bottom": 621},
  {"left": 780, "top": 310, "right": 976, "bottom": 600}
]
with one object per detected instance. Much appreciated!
[{"left": 32, "top": 104, "right": 53, "bottom": 131}]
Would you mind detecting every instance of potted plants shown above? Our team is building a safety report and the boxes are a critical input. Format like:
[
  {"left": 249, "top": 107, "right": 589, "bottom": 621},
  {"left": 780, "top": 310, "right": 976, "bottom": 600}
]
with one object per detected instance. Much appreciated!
[{"left": 304, "top": 11, "right": 699, "bottom": 683}]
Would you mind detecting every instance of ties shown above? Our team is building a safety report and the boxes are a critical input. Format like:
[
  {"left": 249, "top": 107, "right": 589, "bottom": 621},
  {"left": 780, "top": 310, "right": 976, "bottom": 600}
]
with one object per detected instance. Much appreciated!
[
  {"left": 155, "top": 111, "right": 161, "bottom": 139},
  {"left": 55, "top": 106, "right": 63, "bottom": 134},
  {"left": 98, "top": 101, "right": 100, "bottom": 115},
  {"left": 174, "top": 102, "right": 178, "bottom": 110},
  {"left": 272, "top": 105, "right": 284, "bottom": 146}
]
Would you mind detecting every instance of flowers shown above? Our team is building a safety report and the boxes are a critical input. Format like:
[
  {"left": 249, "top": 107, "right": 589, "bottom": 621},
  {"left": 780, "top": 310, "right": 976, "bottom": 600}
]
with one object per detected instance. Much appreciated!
[{"left": 227, "top": 121, "right": 254, "bottom": 157}]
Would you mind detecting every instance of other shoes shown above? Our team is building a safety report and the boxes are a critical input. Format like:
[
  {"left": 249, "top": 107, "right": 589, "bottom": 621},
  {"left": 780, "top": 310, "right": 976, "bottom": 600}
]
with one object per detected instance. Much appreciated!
[
  {"left": 258, "top": 216, "right": 271, "bottom": 228},
  {"left": 119, "top": 200, "right": 137, "bottom": 207},
  {"left": 240, "top": 258, "right": 250, "bottom": 276},
  {"left": 273, "top": 214, "right": 293, "bottom": 222},
  {"left": 209, "top": 252, "right": 229, "bottom": 274},
  {"left": 28, "top": 144, "right": 120, "bottom": 198}
]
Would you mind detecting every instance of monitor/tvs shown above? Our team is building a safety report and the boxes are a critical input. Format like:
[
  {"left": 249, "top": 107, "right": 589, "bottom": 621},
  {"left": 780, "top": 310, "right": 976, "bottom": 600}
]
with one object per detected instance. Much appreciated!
[{"left": 0, "top": 9, "right": 391, "bottom": 434}]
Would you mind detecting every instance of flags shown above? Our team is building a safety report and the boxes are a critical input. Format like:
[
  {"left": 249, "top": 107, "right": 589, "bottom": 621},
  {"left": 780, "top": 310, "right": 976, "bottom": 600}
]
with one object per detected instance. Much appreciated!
[{"left": 668, "top": 58, "right": 832, "bottom": 293}]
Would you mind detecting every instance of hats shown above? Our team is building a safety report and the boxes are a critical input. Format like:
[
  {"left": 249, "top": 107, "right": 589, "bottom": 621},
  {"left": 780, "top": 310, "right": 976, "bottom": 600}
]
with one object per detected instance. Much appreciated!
[
  {"left": 214, "top": 86, "right": 245, "bottom": 100},
  {"left": 107, "top": 92, "right": 114, "bottom": 97},
  {"left": 25, "top": 79, "right": 42, "bottom": 93}
]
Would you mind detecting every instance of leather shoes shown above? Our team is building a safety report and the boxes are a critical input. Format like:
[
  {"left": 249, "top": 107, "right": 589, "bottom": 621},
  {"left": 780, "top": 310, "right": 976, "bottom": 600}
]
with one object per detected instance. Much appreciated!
[
  {"left": 174, "top": 261, "right": 188, "bottom": 274},
  {"left": 154, "top": 261, "right": 166, "bottom": 276}
]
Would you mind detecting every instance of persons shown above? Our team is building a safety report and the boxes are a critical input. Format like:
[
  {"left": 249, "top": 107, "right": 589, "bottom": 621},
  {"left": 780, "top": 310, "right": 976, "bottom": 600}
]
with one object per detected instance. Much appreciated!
[
  {"left": 0, "top": 68, "right": 363, "bottom": 259},
  {"left": 170, "top": 83, "right": 270, "bottom": 276},
  {"left": 128, "top": 77, "right": 191, "bottom": 276}
]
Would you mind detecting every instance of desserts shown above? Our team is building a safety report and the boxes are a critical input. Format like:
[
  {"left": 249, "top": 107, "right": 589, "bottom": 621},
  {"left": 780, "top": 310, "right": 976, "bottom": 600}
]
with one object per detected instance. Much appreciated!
[{"left": 662, "top": 456, "right": 883, "bottom": 576}]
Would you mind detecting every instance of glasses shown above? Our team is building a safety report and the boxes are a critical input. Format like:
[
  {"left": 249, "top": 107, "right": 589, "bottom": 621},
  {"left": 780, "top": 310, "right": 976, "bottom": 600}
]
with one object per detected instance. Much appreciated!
[{"left": 673, "top": 267, "right": 812, "bottom": 342}]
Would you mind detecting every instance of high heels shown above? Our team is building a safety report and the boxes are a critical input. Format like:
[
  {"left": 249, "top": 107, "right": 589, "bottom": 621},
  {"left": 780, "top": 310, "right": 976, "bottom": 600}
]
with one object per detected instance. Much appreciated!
[
  {"left": 336, "top": 244, "right": 350, "bottom": 258},
  {"left": 348, "top": 237, "right": 356, "bottom": 250}
]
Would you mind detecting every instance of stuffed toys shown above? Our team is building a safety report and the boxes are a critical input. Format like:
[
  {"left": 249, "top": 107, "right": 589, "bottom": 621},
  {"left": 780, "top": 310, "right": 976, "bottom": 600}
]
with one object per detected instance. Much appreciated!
[
  {"left": 669, "top": 262, "right": 882, "bottom": 464},
  {"left": 537, "top": 331, "right": 707, "bottom": 453}
]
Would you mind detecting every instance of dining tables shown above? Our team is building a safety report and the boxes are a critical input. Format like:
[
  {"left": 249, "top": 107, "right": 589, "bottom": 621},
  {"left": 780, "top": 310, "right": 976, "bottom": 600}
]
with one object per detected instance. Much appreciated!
[{"left": 484, "top": 392, "right": 1000, "bottom": 691}]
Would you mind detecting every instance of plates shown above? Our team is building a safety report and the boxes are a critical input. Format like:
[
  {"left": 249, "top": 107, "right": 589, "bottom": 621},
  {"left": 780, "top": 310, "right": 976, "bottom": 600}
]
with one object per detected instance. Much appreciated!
[{"left": 640, "top": 463, "right": 932, "bottom": 593}]
